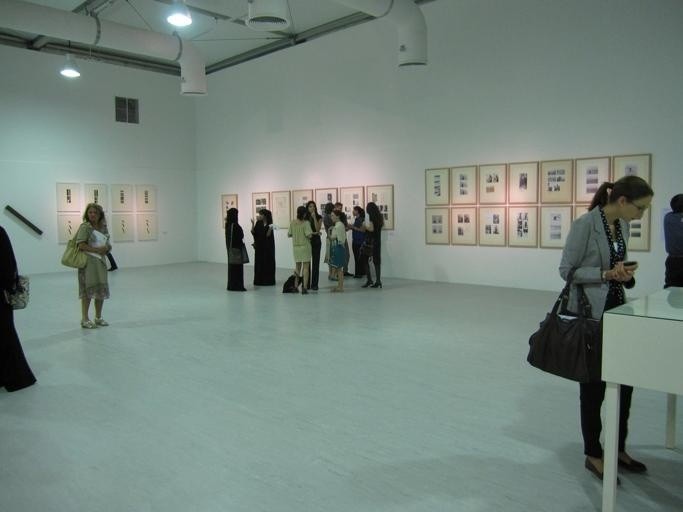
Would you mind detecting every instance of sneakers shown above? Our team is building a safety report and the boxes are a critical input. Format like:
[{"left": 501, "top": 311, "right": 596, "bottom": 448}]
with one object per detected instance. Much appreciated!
[
  {"left": 292, "top": 286, "right": 345, "bottom": 294},
  {"left": 328, "top": 272, "right": 364, "bottom": 281}
]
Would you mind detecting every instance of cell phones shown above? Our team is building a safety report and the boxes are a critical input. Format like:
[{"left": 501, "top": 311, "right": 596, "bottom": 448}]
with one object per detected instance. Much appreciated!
[{"left": 623, "top": 261, "right": 637, "bottom": 266}]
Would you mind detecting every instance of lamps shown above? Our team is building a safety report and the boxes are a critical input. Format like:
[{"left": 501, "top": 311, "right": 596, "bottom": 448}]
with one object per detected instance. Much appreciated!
[
  {"left": 59, "top": 52, "right": 81, "bottom": 78},
  {"left": 166, "top": 0, "right": 194, "bottom": 27}
]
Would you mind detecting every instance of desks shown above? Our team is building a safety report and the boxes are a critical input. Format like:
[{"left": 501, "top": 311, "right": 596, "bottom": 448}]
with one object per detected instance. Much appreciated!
[{"left": 601, "top": 285, "right": 683, "bottom": 512}]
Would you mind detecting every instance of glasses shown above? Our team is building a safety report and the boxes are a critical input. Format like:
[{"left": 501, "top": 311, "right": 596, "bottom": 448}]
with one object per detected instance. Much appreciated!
[{"left": 629, "top": 200, "right": 646, "bottom": 213}]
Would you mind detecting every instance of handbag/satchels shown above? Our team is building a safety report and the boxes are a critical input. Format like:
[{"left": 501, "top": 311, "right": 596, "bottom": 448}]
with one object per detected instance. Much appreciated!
[
  {"left": 61, "top": 238, "right": 87, "bottom": 268},
  {"left": 358, "top": 232, "right": 375, "bottom": 256},
  {"left": 528, "top": 266, "right": 601, "bottom": 386},
  {"left": 227, "top": 246, "right": 250, "bottom": 265},
  {"left": 283, "top": 272, "right": 301, "bottom": 292},
  {"left": 6, "top": 277, "right": 30, "bottom": 308}
]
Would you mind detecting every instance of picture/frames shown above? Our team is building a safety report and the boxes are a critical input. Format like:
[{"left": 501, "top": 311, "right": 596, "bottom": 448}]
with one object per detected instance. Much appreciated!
[
  {"left": 55, "top": 182, "right": 159, "bottom": 245},
  {"left": 424, "top": 152, "right": 652, "bottom": 253},
  {"left": 220, "top": 184, "right": 395, "bottom": 231}
]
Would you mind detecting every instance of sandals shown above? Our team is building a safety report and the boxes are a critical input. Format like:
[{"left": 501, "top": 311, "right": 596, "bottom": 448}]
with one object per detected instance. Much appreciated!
[
  {"left": 81, "top": 320, "right": 98, "bottom": 329},
  {"left": 95, "top": 318, "right": 108, "bottom": 326}
]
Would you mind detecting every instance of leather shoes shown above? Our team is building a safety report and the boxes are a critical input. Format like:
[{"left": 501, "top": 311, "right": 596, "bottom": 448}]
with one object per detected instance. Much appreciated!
[
  {"left": 108, "top": 266, "right": 117, "bottom": 271},
  {"left": 616, "top": 456, "right": 647, "bottom": 473},
  {"left": 586, "top": 456, "right": 621, "bottom": 485}
]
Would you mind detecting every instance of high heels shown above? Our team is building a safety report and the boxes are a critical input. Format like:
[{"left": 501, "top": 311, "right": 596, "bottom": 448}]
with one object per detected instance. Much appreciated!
[
  {"left": 362, "top": 281, "right": 373, "bottom": 288},
  {"left": 371, "top": 282, "right": 382, "bottom": 288}
]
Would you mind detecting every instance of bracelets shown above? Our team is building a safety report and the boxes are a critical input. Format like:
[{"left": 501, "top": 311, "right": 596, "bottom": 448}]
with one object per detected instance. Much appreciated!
[{"left": 602, "top": 270, "right": 607, "bottom": 282}]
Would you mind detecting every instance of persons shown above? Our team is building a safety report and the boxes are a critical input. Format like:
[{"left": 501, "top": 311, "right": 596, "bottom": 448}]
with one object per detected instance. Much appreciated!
[
  {"left": 250, "top": 209, "right": 276, "bottom": 286},
  {"left": 106, "top": 252, "right": 118, "bottom": 271},
  {"left": 225, "top": 208, "right": 246, "bottom": 291},
  {"left": 0, "top": 224, "right": 37, "bottom": 391},
  {"left": 287, "top": 200, "right": 384, "bottom": 295},
  {"left": 75, "top": 203, "right": 110, "bottom": 329},
  {"left": 663, "top": 193, "right": 683, "bottom": 288},
  {"left": 558, "top": 175, "right": 655, "bottom": 485}
]
